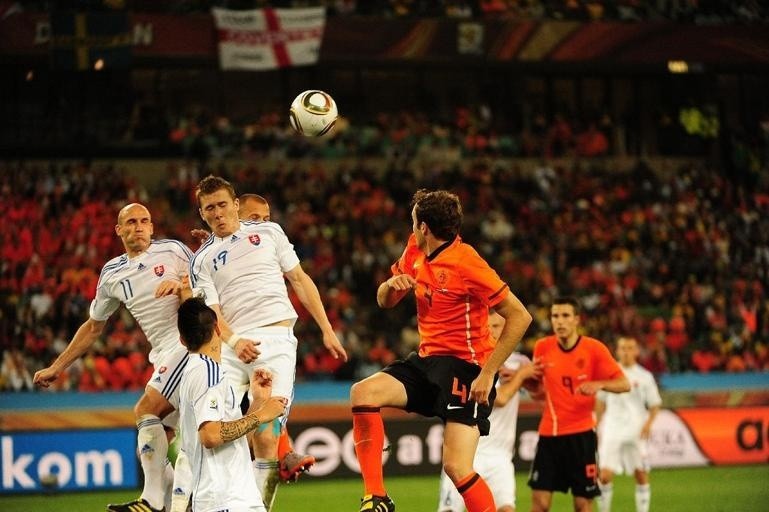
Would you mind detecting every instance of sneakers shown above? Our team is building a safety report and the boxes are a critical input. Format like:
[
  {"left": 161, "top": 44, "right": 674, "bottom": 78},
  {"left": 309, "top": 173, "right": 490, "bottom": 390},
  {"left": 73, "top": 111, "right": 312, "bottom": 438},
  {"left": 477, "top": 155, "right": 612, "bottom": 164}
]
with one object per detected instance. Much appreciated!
[
  {"left": 360, "top": 492, "right": 394, "bottom": 512},
  {"left": 280, "top": 451, "right": 315, "bottom": 483},
  {"left": 107, "top": 498, "right": 165, "bottom": 512}
]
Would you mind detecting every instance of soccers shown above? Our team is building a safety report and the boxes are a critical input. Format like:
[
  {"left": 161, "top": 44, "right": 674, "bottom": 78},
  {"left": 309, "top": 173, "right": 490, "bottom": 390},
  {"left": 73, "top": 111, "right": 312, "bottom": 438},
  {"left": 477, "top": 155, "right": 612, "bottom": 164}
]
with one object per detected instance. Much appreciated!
[{"left": 290, "top": 90, "right": 338, "bottom": 137}]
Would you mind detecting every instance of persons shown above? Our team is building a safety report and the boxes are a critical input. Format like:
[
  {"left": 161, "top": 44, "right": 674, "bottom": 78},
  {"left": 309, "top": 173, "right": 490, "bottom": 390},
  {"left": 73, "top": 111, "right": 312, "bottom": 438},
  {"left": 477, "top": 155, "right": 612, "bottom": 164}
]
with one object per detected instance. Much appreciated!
[
  {"left": 187, "top": 192, "right": 315, "bottom": 484},
  {"left": 436, "top": 308, "right": 546, "bottom": 512},
  {"left": 589, "top": 335, "right": 664, "bottom": 512},
  {"left": 344, "top": 186, "right": 534, "bottom": 512},
  {"left": 2, "top": 2, "right": 769, "bottom": 394},
  {"left": 491, "top": 297, "right": 633, "bottom": 512},
  {"left": 184, "top": 172, "right": 350, "bottom": 510},
  {"left": 31, "top": 201, "right": 198, "bottom": 511},
  {"left": 174, "top": 292, "right": 291, "bottom": 511}
]
